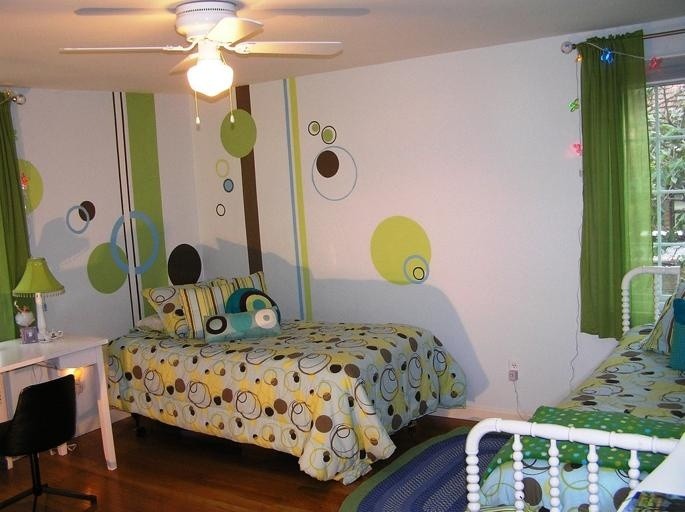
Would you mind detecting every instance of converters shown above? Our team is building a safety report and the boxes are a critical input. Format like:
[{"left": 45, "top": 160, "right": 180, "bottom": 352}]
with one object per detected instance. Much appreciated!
[{"left": 508, "top": 370, "right": 518, "bottom": 381}]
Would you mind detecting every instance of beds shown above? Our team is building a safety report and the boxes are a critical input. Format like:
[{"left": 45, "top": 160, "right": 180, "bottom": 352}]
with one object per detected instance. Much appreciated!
[
  {"left": 464, "top": 265, "right": 685, "bottom": 511},
  {"left": 104, "top": 321, "right": 442, "bottom": 486}
]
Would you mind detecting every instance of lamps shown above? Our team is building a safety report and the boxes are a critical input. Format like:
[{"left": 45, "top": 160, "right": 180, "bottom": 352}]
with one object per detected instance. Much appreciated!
[
  {"left": 12, "top": 257, "right": 66, "bottom": 342},
  {"left": 184, "top": 40, "right": 236, "bottom": 126}
]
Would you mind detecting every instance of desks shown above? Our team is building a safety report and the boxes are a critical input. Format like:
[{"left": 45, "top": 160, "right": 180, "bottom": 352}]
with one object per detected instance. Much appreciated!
[{"left": 0, "top": 335, "right": 119, "bottom": 472}]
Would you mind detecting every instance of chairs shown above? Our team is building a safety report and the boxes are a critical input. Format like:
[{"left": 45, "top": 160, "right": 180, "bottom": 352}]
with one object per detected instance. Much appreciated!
[{"left": 0, "top": 374, "right": 97, "bottom": 511}]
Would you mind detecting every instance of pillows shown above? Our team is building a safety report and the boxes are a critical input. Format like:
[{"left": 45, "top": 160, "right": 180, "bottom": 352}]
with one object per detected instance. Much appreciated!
[
  {"left": 133, "top": 269, "right": 281, "bottom": 345},
  {"left": 640, "top": 274, "right": 685, "bottom": 371}
]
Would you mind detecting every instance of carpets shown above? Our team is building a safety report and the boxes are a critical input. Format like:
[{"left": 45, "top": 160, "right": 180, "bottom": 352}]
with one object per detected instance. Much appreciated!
[{"left": 336, "top": 424, "right": 512, "bottom": 512}]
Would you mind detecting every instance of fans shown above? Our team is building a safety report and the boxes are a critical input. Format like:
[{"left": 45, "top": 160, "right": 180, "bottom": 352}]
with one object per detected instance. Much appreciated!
[{"left": 59, "top": 1, "right": 344, "bottom": 73}]
